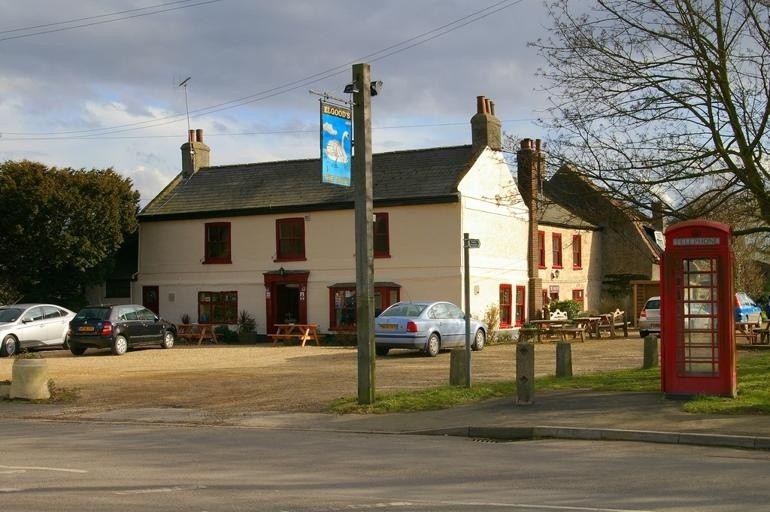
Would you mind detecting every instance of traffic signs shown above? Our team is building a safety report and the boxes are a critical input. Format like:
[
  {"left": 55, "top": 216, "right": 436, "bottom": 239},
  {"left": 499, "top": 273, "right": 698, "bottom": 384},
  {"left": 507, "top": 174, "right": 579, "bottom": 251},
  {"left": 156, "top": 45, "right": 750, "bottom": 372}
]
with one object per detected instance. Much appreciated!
[{"left": 468, "top": 238, "right": 481, "bottom": 248}]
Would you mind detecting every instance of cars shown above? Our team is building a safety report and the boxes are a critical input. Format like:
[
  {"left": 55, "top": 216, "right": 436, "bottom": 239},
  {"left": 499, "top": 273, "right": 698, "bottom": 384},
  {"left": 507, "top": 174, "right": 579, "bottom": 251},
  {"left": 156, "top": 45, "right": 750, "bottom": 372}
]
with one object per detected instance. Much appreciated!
[
  {"left": 67, "top": 303, "right": 177, "bottom": 356},
  {"left": 638, "top": 296, "right": 695, "bottom": 338},
  {"left": 0, "top": 302, "right": 79, "bottom": 357},
  {"left": 705, "top": 291, "right": 763, "bottom": 334},
  {"left": 374, "top": 301, "right": 488, "bottom": 357}
]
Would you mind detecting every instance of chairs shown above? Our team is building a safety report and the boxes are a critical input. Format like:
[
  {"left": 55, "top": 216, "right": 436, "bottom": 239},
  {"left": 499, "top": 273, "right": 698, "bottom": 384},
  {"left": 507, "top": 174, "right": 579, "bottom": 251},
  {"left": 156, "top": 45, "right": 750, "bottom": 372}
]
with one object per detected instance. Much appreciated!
[{"left": 753, "top": 320, "right": 770, "bottom": 345}]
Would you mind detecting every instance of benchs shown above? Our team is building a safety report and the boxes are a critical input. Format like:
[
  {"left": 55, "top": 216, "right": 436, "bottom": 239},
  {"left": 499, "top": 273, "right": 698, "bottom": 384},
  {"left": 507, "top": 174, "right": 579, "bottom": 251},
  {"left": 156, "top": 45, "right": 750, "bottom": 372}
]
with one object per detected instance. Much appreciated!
[
  {"left": 574, "top": 307, "right": 629, "bottom": 339},
  {"left": 267, "top": 334, "right": 326, "bottom": 346},
  {"left": 177, "top": 332, "right": 225, "bottom": 344}
]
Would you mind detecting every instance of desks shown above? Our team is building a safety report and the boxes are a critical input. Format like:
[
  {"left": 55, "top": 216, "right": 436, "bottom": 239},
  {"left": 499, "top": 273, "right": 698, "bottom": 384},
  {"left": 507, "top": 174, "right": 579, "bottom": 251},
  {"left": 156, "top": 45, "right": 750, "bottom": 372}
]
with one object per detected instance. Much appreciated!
[
  {"left": 272, "top": 323, "right": 321, "bottom": 347},
  {"left": 529, "top": 316, "right": 602, "bottom": 342},
  {"left": 178, "top": 322, "right": 218, "bottom": 345},
  {"left": 735, "top": 321, "right": 760, "bottom": 344}
]
